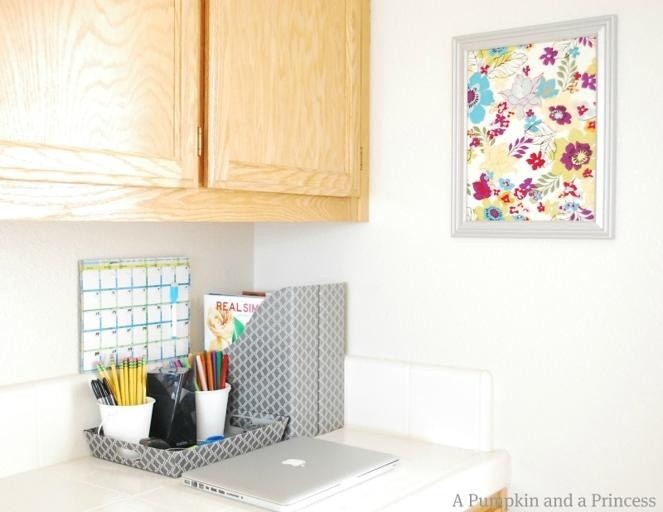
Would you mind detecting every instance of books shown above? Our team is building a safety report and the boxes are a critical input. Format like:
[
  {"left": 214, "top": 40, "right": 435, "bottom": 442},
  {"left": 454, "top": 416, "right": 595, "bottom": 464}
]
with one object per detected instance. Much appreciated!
[
  {"left": 201, "top": 290, "right": 274, "bottom": 352},
  {"left": 146, "top": 372, "right": 185, "bottom": 440}
]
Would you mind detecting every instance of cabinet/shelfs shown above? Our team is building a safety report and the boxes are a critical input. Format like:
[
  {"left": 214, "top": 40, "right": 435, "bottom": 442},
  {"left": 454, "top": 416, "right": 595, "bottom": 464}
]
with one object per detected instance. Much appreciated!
[{"left": 1, "top": 0, "right": 371, "bottom": 226}]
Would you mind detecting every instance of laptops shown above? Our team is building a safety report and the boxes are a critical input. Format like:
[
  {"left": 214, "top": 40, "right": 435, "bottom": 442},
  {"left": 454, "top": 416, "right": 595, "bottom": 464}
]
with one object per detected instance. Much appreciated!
[{"left": 181, "top": 435, "right": 400, "bottom": 512}]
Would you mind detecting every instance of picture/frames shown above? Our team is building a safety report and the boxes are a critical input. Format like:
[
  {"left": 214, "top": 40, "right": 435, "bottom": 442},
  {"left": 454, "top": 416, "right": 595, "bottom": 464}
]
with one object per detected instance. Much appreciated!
[{"left": 445, "top": 12, "right": 620, "bottom": 242}]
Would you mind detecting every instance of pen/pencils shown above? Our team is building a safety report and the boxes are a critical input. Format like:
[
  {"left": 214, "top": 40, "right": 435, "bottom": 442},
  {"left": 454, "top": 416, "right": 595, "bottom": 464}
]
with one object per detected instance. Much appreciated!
[
  {"left": 91, "top": 354, "right": 148, "bottom": 406},
  {"left": 163, "top": 350, "right": 229, "bottom": 392}
]
